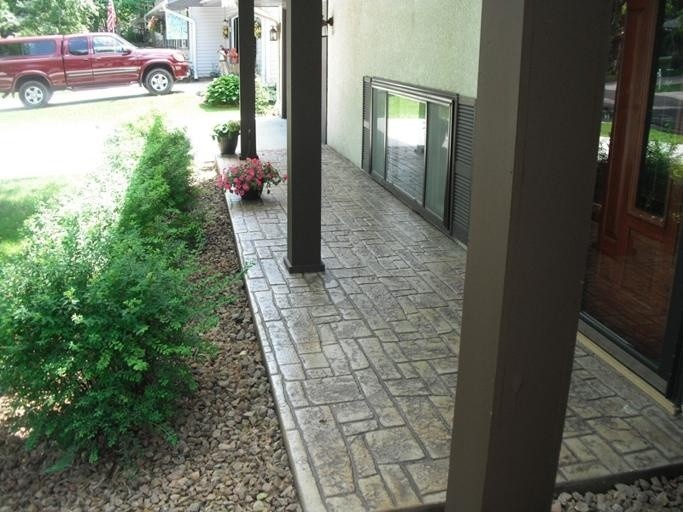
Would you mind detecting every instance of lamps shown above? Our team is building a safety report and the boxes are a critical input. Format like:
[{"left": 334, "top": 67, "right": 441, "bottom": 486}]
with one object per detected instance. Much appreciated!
[{"left": 269, "top": 25, "right": 279, "bottom": 41}]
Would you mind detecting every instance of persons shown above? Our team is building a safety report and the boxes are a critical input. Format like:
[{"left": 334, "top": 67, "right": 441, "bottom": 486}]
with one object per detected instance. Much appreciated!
[{"left": 216, "top": 44, "right": 229, "bottom": 76}]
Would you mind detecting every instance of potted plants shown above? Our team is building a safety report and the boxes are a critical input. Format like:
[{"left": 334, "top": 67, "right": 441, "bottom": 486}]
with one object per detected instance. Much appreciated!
[{"left": 211, "top": 119, "right": 242, "bottom": 158}]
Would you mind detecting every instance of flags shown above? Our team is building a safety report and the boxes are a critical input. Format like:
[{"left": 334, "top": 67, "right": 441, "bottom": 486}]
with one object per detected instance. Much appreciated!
[{"left": 105, "top": 0, "right": 116, "bottom": 32}]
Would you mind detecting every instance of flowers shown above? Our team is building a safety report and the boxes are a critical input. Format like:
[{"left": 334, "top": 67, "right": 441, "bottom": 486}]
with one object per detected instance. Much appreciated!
[{"left": 216, "top": 156, "right": 286, "bottom": 191}]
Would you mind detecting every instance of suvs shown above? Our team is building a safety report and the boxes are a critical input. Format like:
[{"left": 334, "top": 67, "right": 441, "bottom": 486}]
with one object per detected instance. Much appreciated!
[{"left": 0, "top": 31, "right": 190, "bottom": 109}]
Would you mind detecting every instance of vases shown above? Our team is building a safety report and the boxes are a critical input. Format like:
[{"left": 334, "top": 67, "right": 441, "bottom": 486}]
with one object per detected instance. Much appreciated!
[{"left": 238, "top": 188, "right": 264, "bottom": 199}]
